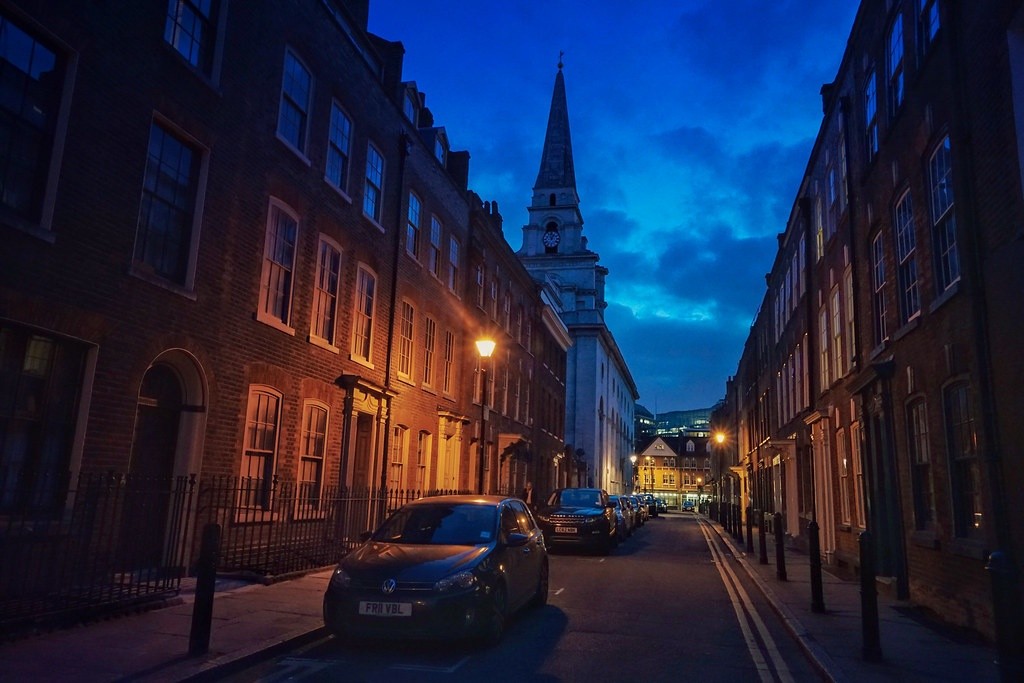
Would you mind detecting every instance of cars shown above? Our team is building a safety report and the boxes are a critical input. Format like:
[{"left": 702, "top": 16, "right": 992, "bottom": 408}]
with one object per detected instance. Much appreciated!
[
  {"left": 619, "top": 495, "right": 636, "bottom": 528},
  {"left": 656, "top": 498, "right": 667, "bottom": 513},
  {"left": 628, "top": 496, "right": 644, "bottom": 526},
  {"left": 681, "top": 501, "right": 695, "bottom": 512},
  {"left": 609, "top": 495, "right": 626, "bottom": 532},
  {"left": 321, "top": 495, "right": 549, "bottom": 649},
  {"left": 643, "top": 494, "right": 658, "bottom": 518},
  {"left": 636, "top": 494, "right": 650, "bottom": 521}
]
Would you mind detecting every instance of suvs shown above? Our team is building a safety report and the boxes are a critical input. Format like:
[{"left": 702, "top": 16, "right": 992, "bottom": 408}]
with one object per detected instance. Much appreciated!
[{"left": 537, "top": 487, "right": 617, "bottom": 551}]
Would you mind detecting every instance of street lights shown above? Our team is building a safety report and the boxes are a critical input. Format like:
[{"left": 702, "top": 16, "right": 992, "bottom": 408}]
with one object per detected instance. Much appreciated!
[
  {"left": 629, "top": 453, "right": 637, "bottom": 494},
  {"left": 716, "top": 431, "right": 726, "bottom": 527},
  {"left": 475, "top": 332, "right": 496, "bottom": 493},
  {"left": 698, "top": 478, "right": 702, "bottom": 513}
]
